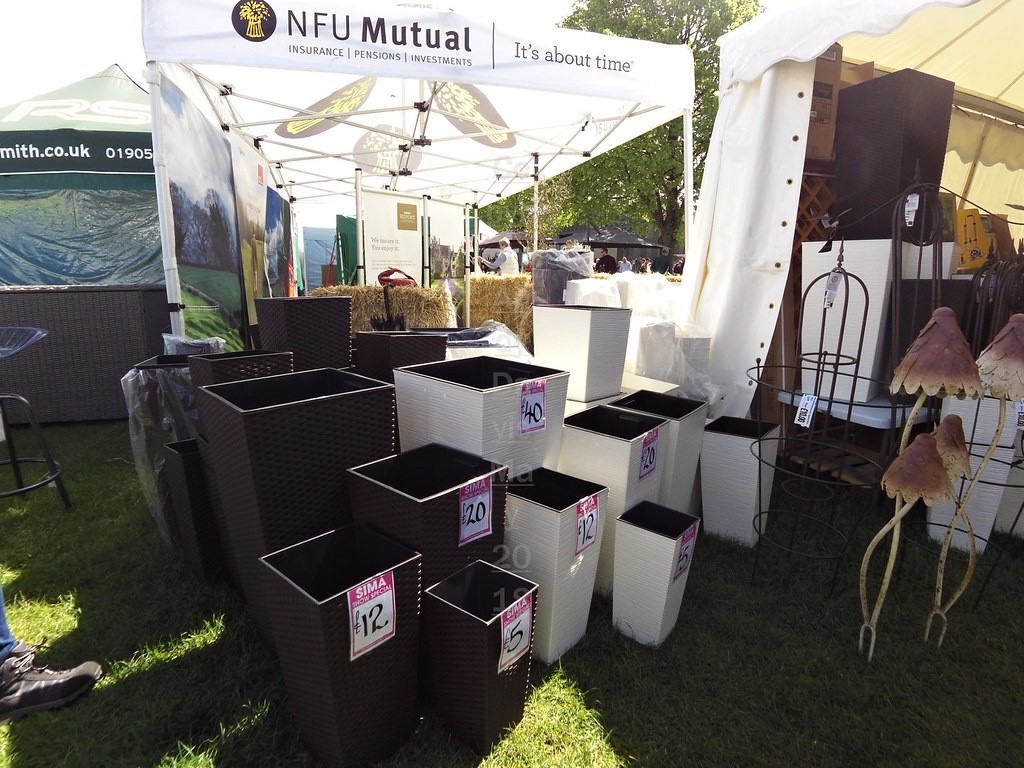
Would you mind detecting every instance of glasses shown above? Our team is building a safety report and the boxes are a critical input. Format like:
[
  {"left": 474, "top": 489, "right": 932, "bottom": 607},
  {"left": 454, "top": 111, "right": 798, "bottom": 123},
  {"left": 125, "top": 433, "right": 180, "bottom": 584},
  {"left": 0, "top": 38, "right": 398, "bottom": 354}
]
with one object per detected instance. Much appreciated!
[{"left": 600, "top": 250, "right": 604, "bottom": 253}]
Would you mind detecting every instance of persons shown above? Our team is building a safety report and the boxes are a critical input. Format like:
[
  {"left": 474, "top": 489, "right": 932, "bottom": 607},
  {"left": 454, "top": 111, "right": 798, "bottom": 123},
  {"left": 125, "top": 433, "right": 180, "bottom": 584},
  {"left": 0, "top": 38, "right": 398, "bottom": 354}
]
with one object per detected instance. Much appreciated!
[
  {"left": 477, "top": 237, "right": 520, "bottom": 276},
  {"left": 594, "top": 247, "right": 683, "bottom": 276},
  {"left": 0, "top": 586, "right": 102, "bottom": 718}
]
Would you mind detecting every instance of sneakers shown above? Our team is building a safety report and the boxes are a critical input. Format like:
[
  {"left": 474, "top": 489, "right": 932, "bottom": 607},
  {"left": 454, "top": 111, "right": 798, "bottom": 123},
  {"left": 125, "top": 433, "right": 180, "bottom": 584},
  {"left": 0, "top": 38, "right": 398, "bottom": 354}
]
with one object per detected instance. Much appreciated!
[{"left": 0, "top": 640, "right": 102, "bottom": 723}]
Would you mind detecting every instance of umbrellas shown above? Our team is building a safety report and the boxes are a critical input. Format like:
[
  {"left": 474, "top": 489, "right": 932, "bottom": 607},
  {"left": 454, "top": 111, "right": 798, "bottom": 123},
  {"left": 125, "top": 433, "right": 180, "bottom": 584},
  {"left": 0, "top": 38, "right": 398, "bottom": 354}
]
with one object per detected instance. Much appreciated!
[{"left": 369, "top": 282, "right": 406, "bottom": 331}]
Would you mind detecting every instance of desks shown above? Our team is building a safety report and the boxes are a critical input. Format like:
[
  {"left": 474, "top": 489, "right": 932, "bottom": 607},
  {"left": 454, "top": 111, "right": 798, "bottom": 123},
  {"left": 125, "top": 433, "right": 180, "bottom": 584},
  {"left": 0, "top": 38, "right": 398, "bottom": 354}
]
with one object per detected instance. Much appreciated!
[
  {"left": 779, "top": 389, "right": 940, "bottom": 430},
  {"left": 0, "top": 327, "right": 73, "bottom": 511}
]
